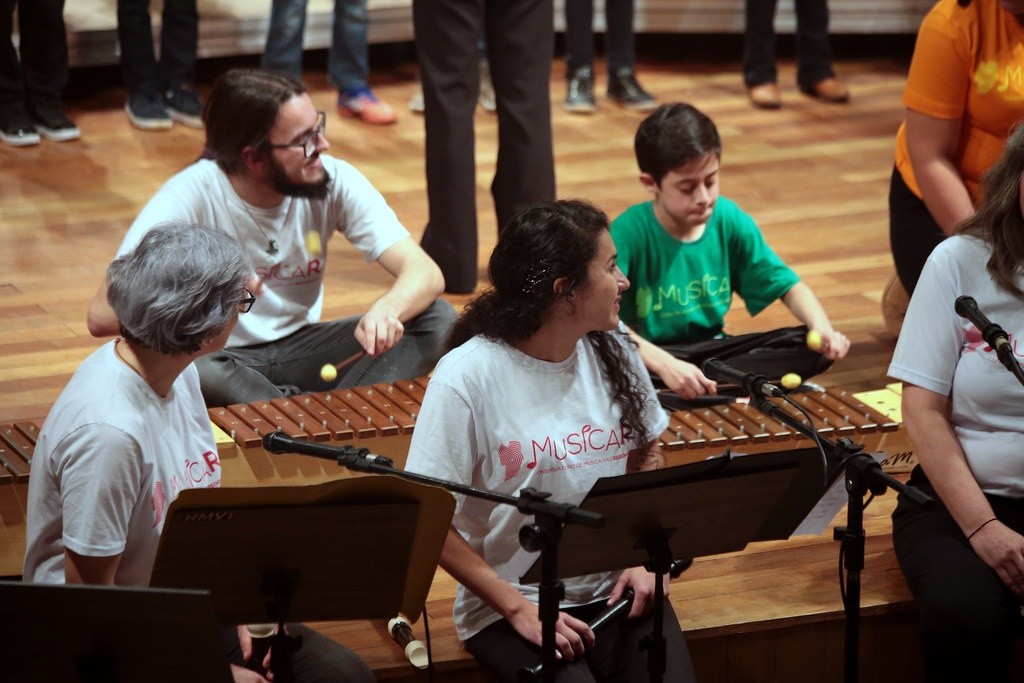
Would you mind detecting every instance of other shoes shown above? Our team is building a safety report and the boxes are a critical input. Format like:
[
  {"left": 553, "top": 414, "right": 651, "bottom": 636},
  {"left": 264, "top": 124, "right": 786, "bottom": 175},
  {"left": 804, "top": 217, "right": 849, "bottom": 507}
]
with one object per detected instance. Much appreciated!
[
  {"left": 565, "top": 63, "right": 596, "bottom": 113},
  {"left": 748, "top": 82, "right": 782, "bottom": 109},
  {"left": 125, "top": 93, "right": 175, "bottom": 131},
  {"left": 880, "top": 273, "right": 912, "bottom": 337},
  {"left": 0, "top": 117, "right": 40, "bottom": 146},
  {"left": 608, "top": 66, "right": 656, "bottom": 111},
  {"left": 161, "top": 85, "right": 204, "bottom": 130},
  {"left": 476, "top": 58, "right": 496, "bottom": 112},
  {"left": 801, "top": 75, "right": 850, "bottom": 102},
  {"left": 408, "top": 89, "right": 426, "bottom": 114},
  {"left": 32, "top": 105, "right": 81, "bottom": 141}
]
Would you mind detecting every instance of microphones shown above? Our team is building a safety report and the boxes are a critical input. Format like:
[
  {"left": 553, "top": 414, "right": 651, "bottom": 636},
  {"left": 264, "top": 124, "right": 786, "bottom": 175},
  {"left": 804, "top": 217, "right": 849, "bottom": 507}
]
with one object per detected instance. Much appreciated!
[
  {"left": 262, "top": 432, "right": 392, "bottom": 466},
  {"left": 701, "top": 358, "right": 782, "bottom": 398},
  {"left": 955, "top": 295, "right": 1013, "bottom": 355}
]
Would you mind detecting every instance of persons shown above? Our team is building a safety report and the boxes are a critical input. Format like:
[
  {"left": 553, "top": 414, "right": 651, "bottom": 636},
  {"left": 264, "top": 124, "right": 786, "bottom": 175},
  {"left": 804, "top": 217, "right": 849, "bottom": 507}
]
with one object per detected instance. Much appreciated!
[
  {"left": 400, "top": 197, "right": 693, "bottom": 683},
  {"left": 115, "top": 0, "right": 204, "bottom": 131},
  {"left": 0, "top": 0, "right": 80, "bottom": 147},
  {"left": 411, "top": 0, "right": 556, "bottom": 295},
  {"left": 608, "top": 104, "right": 851, "bottom": 401},
  {"left": 562, "top": 0, "right": 659, "bottom": 114},
  {"left": 883, "top": 1, "right": 1024, "bottom": 336},
  {"left": 741, "top": 1, "right": 852, "bottom": 111},
  {"left": 25, "top": 220, "right": 372, "bottom": 683},
  {"left": 261, "top": 0, "right": 396, "bottom": 125},
  {"left": 891, "top": 125, "right": 1024, "bottom": 683},
  {"left": 85, "top": 69, "right": 459, "bottom": 412}
]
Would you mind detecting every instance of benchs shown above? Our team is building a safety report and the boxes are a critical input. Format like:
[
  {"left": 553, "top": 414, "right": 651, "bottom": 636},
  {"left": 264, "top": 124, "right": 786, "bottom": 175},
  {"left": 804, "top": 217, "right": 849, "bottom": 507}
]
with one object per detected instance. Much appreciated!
[{"left": 303, "top": 534, "right": 919, "bottom": 683}]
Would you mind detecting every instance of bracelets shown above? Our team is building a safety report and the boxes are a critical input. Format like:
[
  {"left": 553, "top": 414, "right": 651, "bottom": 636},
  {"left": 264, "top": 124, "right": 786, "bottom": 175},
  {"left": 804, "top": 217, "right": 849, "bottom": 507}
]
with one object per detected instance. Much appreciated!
[{"left": 968, "top": 518, "right": 1000, "bottom": 540}]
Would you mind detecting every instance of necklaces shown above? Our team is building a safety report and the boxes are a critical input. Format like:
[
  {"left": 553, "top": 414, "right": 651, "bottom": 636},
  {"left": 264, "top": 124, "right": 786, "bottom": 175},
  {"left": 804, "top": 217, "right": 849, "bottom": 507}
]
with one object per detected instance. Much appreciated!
[
  {"left": 115, "top": 337, "right": 169, "bottom": 399},
  {"left": 241, "top": 198, "right": 293, "bottom": 254}
]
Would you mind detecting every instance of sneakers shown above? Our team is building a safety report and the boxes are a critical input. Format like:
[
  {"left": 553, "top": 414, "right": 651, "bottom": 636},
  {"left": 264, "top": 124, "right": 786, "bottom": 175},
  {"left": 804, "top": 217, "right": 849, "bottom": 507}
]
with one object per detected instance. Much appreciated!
[{"left": 337, "top": 88, "right": 397, "bottom": 124}]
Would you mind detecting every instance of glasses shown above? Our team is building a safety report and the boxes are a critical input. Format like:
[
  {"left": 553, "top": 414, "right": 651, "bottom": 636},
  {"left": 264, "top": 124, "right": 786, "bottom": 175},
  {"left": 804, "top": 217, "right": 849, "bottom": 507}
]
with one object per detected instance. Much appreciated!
[
  {"left": 266, "top": 111, "right": 326, "bottom": 157},
  {"left": 221, "top": 288, "right": 256, "bottom": 314}
]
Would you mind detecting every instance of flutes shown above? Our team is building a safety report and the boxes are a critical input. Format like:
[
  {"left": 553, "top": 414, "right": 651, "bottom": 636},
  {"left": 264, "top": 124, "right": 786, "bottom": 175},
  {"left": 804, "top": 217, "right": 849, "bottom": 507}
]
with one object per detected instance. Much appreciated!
[
  {"left": 517, "top": 558, "right": 694, "bottom": 683},
  {"left": 386, "top": 616, "right": 429, "bottom": 670}
]
